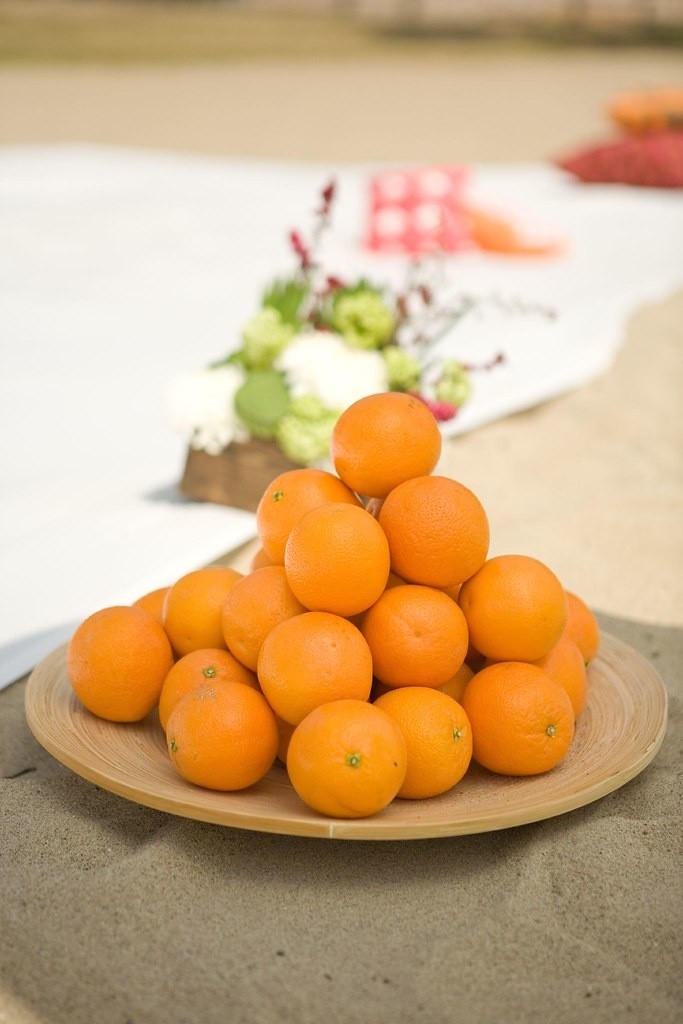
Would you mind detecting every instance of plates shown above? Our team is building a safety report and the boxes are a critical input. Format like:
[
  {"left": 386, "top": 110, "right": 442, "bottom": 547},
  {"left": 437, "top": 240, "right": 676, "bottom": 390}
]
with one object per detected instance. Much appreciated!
[{"left": 24, "top": 625, "right": 669, "bottom": 840}]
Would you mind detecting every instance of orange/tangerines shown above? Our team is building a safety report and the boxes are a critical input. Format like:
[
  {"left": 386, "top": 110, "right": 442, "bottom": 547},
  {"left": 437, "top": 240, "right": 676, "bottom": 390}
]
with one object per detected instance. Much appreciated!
[{"left": 68, "top": 391, "right": 599, "bottom": 818}]
